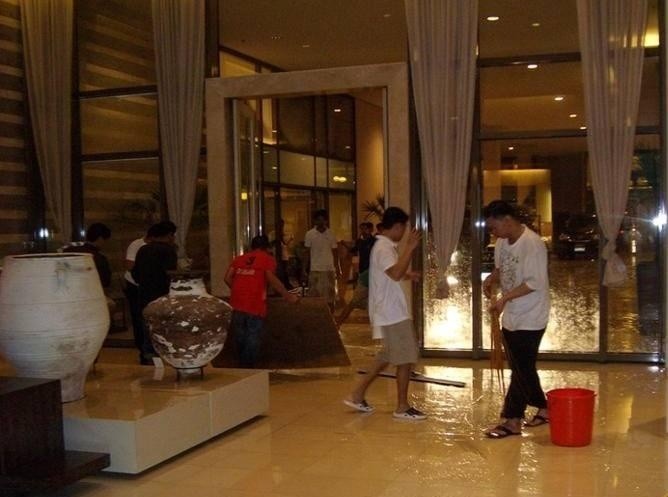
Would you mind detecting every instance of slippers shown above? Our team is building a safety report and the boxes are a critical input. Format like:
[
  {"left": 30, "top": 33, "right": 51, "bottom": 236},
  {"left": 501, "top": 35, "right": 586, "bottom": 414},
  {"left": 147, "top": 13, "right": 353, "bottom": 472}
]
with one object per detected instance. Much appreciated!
[
  {"left": 392, "top": 407, "right": 428, "bottom": 419},
  {"left": 343, "top": 399, "right": 376, "bottom": 412},
  {"left": 524, "top": 415, "right": 549, "bottom": 427},
  {"left": 485, "top": 426, "right": 522, "bottom": 438}
]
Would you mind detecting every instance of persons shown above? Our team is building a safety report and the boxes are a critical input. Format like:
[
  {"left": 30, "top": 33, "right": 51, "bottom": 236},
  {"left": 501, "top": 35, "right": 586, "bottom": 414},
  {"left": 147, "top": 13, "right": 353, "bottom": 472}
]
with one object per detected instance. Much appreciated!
[
  {"left": 342, "top": 206, "right": 429, "bottom": 420},
  {"left": 224, "top": 235, "right": 300, "bottom": 368},
  {"left": 482, "top": 200, "right": 550, "bottom": 439},
  {"left": 131, "top": 220, "right": 177, "bottom": 307},
  {"left": 62, "top": 221, "right": 111, "bottom": 287},
  {"left": 123, "top": 223, "right": 160, "bottom": 365},
  {"left": 268, "top": 208, "right": 383, "bottom": 314}
]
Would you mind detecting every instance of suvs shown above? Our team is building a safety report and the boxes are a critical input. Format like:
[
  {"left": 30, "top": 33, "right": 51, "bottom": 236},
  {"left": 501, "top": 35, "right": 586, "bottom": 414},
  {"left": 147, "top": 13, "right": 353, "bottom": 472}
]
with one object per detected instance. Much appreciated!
[{"left": 553, "top": 210, "right": 626, "bottom": 262}]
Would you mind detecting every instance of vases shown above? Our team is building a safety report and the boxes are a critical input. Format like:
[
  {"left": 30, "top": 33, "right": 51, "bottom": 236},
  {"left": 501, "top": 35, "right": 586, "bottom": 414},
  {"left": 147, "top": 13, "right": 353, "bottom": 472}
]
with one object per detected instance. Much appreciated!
[
  {"left": 142, "top": 270, "right": 234, "bottom": 369},
  {"left": 0, "top": 252, "right": 112, "bottom": 404}
]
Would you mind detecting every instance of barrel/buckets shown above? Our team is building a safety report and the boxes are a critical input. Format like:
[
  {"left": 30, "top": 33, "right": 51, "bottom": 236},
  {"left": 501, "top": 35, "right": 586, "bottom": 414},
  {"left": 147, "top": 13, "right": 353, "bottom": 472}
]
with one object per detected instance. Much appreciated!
[{"left": 545, "top": 387, "right": 598, "bottom": 448}]
